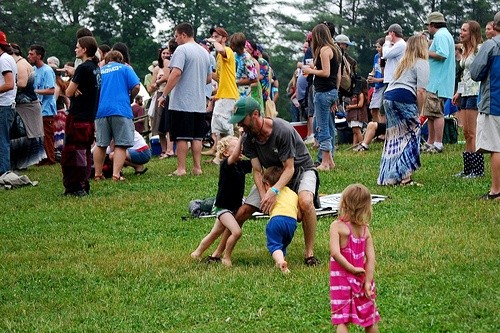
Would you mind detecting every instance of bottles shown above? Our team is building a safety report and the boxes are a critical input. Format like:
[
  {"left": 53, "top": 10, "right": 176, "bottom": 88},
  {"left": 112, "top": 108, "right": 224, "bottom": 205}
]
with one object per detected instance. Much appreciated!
[
  {"left": 292, "top": 98, "right": 299, "bottom": 107},
  {"left": 369, "top": 73, "right": 373, "bottom": 87}
]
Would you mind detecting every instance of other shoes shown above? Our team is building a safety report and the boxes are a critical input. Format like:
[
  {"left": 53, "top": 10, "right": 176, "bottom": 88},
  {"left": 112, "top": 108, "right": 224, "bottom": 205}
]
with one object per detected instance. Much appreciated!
[
  {"left": 309, "top": 144, "right": 320, "bottom": 150},
  {"left": 425, "top": 145, "right": 443, "bottom": 154},
  {"left": 354, "top": 144, "right": 369, "bottom": 152},
  {"left": 419, "top": 143, "right": 432, "bottom": 153}
]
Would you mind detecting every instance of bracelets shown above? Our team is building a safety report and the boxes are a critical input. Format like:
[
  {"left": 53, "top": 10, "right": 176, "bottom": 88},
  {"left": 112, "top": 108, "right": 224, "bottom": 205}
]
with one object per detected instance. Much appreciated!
[{"left": 269, "top": 185, "right": 281, "bottom": 194}]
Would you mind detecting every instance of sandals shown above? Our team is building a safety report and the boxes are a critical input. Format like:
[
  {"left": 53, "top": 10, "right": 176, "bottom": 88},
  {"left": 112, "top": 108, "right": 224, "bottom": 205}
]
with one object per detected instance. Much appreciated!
[
  {"left": 206, "top": 253, "right": 223, "bottom": 261},
  {"left": 158, "top": 151, "right": 175, "bottom": 159},
  {"left": 94, "top": 175, "right": 106, "bottom": 182},
  {"left": 113, "top": 176, "right": 126, "bottom": 181},
  {"left": 480, "top": 191, "right": 500, "bottom": 202},
  {"left": 304, "top": 255, "right": 321, "bottom": 267}
]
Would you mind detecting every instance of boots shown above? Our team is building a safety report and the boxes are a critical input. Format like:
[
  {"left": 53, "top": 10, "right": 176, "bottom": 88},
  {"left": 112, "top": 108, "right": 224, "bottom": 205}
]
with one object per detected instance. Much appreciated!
[
  {"left": 452, "top": 151, "right": 475, "bottom": 178},
  {"left": 464, "top": 152, "right": 485, "bottom": 178}
]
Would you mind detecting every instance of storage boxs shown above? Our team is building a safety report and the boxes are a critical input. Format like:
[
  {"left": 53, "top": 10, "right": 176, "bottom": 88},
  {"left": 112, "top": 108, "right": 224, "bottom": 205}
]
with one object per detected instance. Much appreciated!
[
  {"left": 149, "top": 135, "right": 176, "bottom": 156},
  {"left": 290, "top": 121, "right": 308, "bottom": 139}
]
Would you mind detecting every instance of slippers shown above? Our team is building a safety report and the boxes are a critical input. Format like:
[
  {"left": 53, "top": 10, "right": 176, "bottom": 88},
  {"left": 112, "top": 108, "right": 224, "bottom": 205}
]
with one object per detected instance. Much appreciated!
[{"left": 134, "top": 167, "right": 148, "bottom": 176}]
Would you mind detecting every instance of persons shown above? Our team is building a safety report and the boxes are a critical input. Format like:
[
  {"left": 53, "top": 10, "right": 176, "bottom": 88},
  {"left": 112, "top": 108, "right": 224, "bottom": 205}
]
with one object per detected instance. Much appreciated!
[
  {"left": 26, "top": 46, "right": 58, "bottom": 165},
  {"left": 286, "top": 23, "right": 388, "bottom": 152},
  {"left": 424, "top": 10, "right": 457, "bottom": 152},
  {"left": 0, "top": 31, "right": 48, "bottom": 173},
  {"left": 471, "top": 11, "right": 500, "bottom": 201},
  {"left": 191, "top": 127, "right": 260, "bottom": 267},
  {"left": 48, "top": 28, "right": 151, "bottom": 176},
  {"left": 329, "top": 183, "right": 382, "bottom": 333},
  {"left": 380, "top": 23, "right": 408, "bottom": 89},
  {"left": 157, "top": 22, "right": 213, "bottom": 176},
  {"left": 61, "top": 36, "right": 101, "bottom": 197},
  {"left": 377, "top": 31, "right": 430, "bottom": 186},
  {"left": 262, "top": 166, "right": 303, "bottom": 275},
  {"left": 451, "top": 20, "right": 485, "bottom": 179},
  {"left": 93, "top": 51, "right": 141, "bottom": 182},
  {"left": 132, "top": 28, "right": 279, "bottom": 159},
  {"left": 205, "top": 95, "right": 321, "bottom": 266}
]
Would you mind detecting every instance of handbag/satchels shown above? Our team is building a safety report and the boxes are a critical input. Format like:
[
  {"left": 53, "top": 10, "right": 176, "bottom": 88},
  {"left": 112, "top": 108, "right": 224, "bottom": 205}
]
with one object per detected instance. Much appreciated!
[{"left": 341, "top": 66, "right": 352, "bottom": 92}]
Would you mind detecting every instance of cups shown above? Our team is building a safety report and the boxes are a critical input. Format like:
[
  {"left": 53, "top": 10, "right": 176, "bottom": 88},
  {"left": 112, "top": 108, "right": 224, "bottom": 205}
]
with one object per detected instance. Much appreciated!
[
  {"left": 380, "top": 57, "right": 385, "bottom": 67},
  {"left": 302, "top": 66, "right": 310, "bottom": 76},
  {"left": 306, "top": 59, "right": 313, "bottom": 64},
  {"left": 206, "top": 42, "right": 211, "bottom": 46}
]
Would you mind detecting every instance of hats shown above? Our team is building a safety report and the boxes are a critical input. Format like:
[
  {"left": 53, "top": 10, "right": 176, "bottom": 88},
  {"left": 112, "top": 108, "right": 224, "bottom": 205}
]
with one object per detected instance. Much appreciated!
[
  {"left": 424, "top": 12, "right": 446, "bottom": 25},
  {"left": 0, "top": 32, "right": 9, "bottom": 45},
  {"left": 493, "top": 11, "right": 500, "bottom": 32},
  {"left": 334, "top": 34, "right": 351, "bottom": 44},
  {"left": 209, "top": 27, "right": 228, "bottom": 37},
  {"left": 227, "top": 95, "right": 260, "bottom": 124},
  {"left": 307, "top": 32, "right": 312, "bottom": 40},
  {"left": 384, "top": 24, "right": 402, "bottom": 34}
]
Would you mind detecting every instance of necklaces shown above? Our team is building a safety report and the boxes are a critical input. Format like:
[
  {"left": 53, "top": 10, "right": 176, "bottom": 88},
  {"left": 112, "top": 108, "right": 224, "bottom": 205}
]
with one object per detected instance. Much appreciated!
[{"left": 251, "top": 119, "right": 265, "bottom": 138}]
